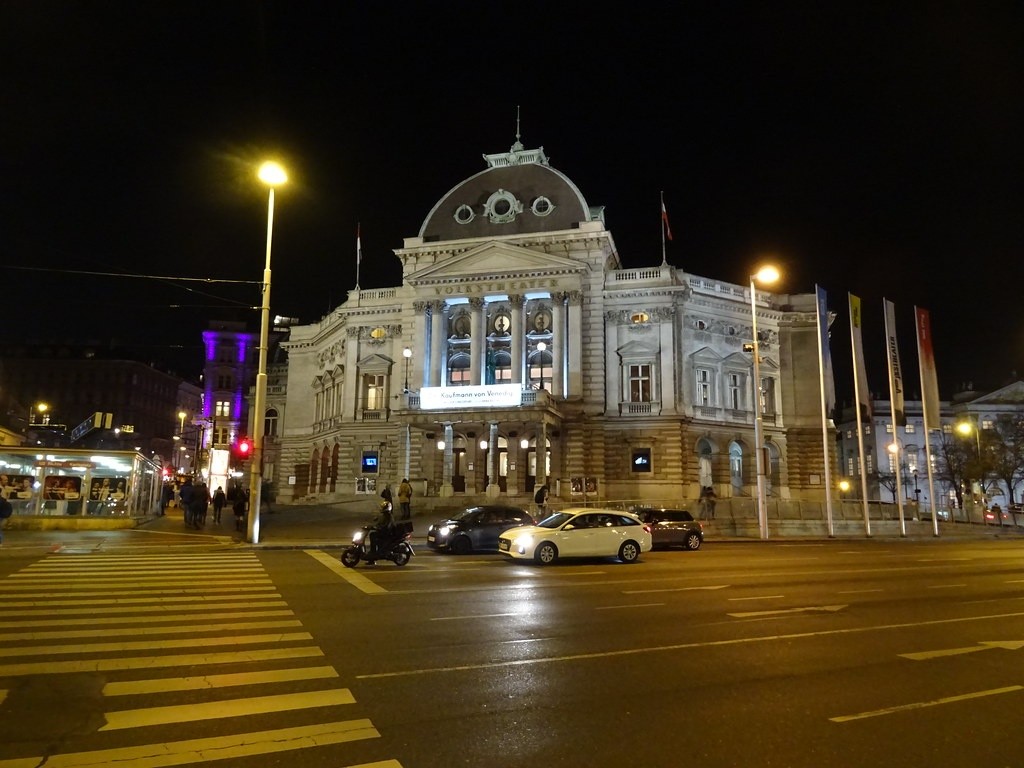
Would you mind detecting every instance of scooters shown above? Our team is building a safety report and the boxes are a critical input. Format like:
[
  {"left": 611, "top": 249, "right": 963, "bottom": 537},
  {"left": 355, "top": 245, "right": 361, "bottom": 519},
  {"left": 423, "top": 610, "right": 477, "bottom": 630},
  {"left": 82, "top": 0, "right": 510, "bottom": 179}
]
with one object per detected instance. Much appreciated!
[{"left": 341, "top": 517, "right": 413, "bottom": 568}]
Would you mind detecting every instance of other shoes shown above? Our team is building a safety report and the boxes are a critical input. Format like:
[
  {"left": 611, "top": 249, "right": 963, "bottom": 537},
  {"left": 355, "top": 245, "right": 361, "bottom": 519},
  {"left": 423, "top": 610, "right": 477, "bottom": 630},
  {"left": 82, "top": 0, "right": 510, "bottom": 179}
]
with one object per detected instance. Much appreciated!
[{"left": 367, "top": 557, "right": 377, "bottom": 565}]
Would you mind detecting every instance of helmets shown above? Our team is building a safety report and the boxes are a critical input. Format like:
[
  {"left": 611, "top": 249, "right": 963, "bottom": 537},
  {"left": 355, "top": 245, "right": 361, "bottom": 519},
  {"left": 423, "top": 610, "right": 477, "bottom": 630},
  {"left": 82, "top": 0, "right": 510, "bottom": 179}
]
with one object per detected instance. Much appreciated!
[{"left": 380, "top": 501, "right": 392, "bottom": 512}]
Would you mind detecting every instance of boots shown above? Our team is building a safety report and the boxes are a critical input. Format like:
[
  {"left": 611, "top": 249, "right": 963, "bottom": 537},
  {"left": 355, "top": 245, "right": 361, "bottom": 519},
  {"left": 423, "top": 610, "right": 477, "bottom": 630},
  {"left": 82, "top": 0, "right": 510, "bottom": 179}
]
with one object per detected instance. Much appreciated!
[
  {"left": 240, "top": 520, "right": 243, "bottom": 531},
  {"left": 236, "top": 520, "right": 239, "bottom": 530}
]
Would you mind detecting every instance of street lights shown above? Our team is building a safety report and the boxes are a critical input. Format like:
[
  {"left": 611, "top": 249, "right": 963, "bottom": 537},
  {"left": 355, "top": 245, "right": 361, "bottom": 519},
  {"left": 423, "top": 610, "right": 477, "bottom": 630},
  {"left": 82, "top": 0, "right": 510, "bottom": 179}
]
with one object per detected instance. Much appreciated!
[
  {"left": 889, "top": 438, "right": 907, "bottom": 499},
  {"left": 247, "top": 162, "right": 287, "bottom": 544},
  {"left": 178, "top": 411, "right": 186, "bottom": 471},
  {"left": 957, "top": 423, "right": 982, "bottom": 502},
  {"left": 912, "top": 469, "right": 918, "bottom": 502},
  {"left": 749, "top": 265, "right": 780, "bottom": 540}
]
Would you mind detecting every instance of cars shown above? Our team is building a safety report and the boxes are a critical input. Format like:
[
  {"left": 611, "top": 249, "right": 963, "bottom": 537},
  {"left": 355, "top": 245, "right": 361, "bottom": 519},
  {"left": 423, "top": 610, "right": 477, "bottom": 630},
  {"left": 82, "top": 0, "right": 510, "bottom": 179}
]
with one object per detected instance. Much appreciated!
[
  {"left": 427, "top": 506, "right": 538, "bottom": 554},
  {"left": 632, "top": 509, "right": 704, "bottom": 551},
  {"left": 498, "top": 507, "right": 653, "bottom": 566}
]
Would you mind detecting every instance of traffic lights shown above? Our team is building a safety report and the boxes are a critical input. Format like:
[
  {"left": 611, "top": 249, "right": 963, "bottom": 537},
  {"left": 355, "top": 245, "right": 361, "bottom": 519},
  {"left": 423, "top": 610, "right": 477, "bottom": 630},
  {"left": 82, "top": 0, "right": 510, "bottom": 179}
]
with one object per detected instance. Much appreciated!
[
  {"left": 238, "top": 440, "right": 250, "bottom": 459},
  {"left": 162, "top": 469, "right": 168, "bottom": 476}
]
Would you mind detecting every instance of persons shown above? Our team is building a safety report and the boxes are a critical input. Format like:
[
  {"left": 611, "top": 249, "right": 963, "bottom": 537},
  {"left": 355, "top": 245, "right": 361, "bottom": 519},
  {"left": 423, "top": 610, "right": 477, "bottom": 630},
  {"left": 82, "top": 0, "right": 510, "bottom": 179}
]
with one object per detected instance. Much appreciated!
[
  {"left": 992, "top": 503, "right": 1001, "bottom": 512},
  {"left": 381, "top": 484, "right": 393, "bottom": 513},
  {"left": 180, "top": 479, "right": 211, "bottom": 528},
  {"left": 262, "top": 479, "right": 274, "bottom": 513},
  {"left": 211, "top": 486, "right": 226, "bottom": 523},
  {"left": 50, "top": 479, "right": 76, "bottom": 499},
  {"left": 535, "top": 486, "right": 549, "bottom": 523},
  {"left": 92, "top": 478, "right": 125, "bottom": 500},
  {"left": 164, "top": 484, "right": 173, "bottom": 507},
  {"left": 232, "top": 481, "right": 246, "bottom": 529},
  {"left": 0, "top": 488, "right": 3, "bottom": 543},
  {"left": 364, "top": 501, "right": 396, "bottom": 567},
  {"left": 0, "top": 475, "right": 32, "bottom": 498},
  {"left": 398, "top": 479, "right": 412, "bottom": 519},
  {"left": 699, "top": 486, "right": 719, "bottom": 518}
]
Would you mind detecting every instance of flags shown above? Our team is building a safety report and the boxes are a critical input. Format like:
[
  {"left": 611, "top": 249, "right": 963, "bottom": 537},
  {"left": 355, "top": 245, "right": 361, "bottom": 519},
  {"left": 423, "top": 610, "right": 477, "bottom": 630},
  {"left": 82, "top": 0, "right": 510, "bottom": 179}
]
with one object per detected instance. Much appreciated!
[
  {"left": 356, "top": 238, "right": 362, "bottom": 264},
  {"left": 663, "top": 204, "right": 672, "bottom": 240}
]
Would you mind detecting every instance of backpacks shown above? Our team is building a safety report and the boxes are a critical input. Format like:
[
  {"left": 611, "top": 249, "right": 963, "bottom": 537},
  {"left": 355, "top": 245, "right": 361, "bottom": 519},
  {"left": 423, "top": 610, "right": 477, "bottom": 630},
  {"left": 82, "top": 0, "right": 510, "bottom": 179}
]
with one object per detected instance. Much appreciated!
[{"left": 215, "top": 492, "right": 224, "bottom": 506}]
